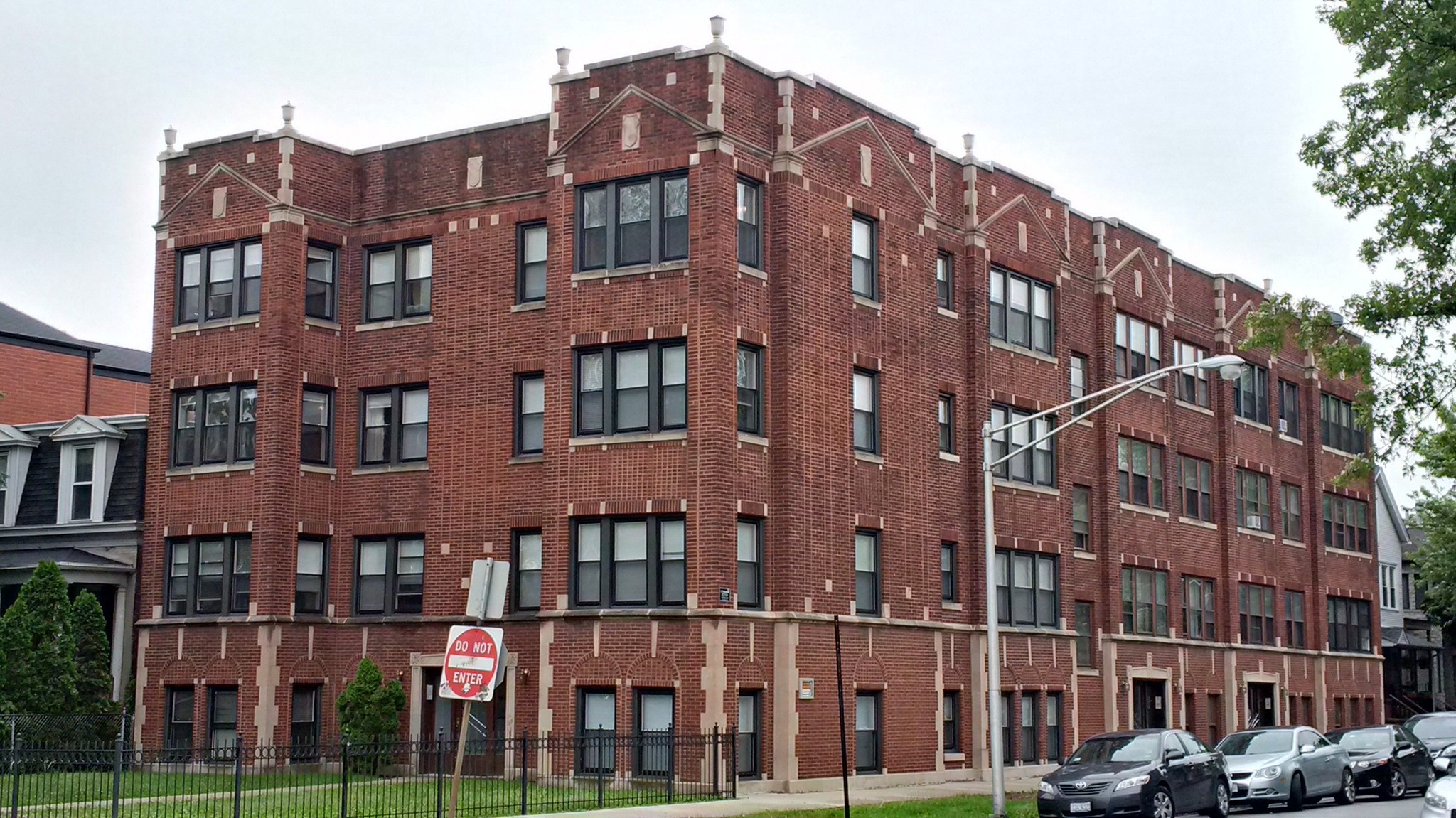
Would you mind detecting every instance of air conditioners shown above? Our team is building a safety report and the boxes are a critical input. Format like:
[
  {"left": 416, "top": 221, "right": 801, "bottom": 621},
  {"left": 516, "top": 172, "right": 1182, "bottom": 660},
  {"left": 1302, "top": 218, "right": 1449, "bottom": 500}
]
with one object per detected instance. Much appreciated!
[
  {"left": 1278, "top": 418, "right": 1288, "bottom": 434},
  {"left": 1246, "top": 514, "right": 1261, "bottom": 530}
]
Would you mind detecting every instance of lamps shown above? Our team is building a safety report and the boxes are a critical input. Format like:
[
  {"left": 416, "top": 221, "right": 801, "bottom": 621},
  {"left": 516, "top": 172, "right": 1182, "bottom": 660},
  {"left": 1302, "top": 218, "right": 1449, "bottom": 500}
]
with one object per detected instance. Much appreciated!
[
  {"left": 519, "top": 668, "right": 530, "bottom": 686},
  {"left": 1120, "top": 679, "right": 1130, "bottom": 693},
  {"left": 1237, "top": 683, "right": 1247, "bottom": 694},
  {"left": 1280, "top": 685, "right": 1289, "bottom": 697},
  {"left": 395, "top": 669, "right": 404, "bottom": 682}
]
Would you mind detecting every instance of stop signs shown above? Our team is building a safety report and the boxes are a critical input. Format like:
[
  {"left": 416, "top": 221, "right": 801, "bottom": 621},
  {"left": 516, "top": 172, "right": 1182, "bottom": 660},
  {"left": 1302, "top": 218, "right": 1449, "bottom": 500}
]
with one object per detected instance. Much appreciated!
[{"left": 444, "top": 629, "right": 497, "bottom": 699}]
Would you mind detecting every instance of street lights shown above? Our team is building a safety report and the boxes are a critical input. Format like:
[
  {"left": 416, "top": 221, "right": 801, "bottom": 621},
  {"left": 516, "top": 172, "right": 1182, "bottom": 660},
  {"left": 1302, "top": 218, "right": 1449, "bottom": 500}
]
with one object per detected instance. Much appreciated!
[{"left": 980, "top": 353, "right": 1247, "bottom": 817}]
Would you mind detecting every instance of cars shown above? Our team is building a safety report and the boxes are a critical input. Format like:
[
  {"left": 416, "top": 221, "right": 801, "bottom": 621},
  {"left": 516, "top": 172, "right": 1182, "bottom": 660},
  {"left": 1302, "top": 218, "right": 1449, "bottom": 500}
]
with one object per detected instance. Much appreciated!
[
  {"left": 1037, "top": 729, "right": 1233, "bottom": 818},
  {"left": 1321, "top": 710, "right": 1456, "bottom": 818},
  {"left": 1215, "top": 724, "right": 1358, "bottom": 812}
]
